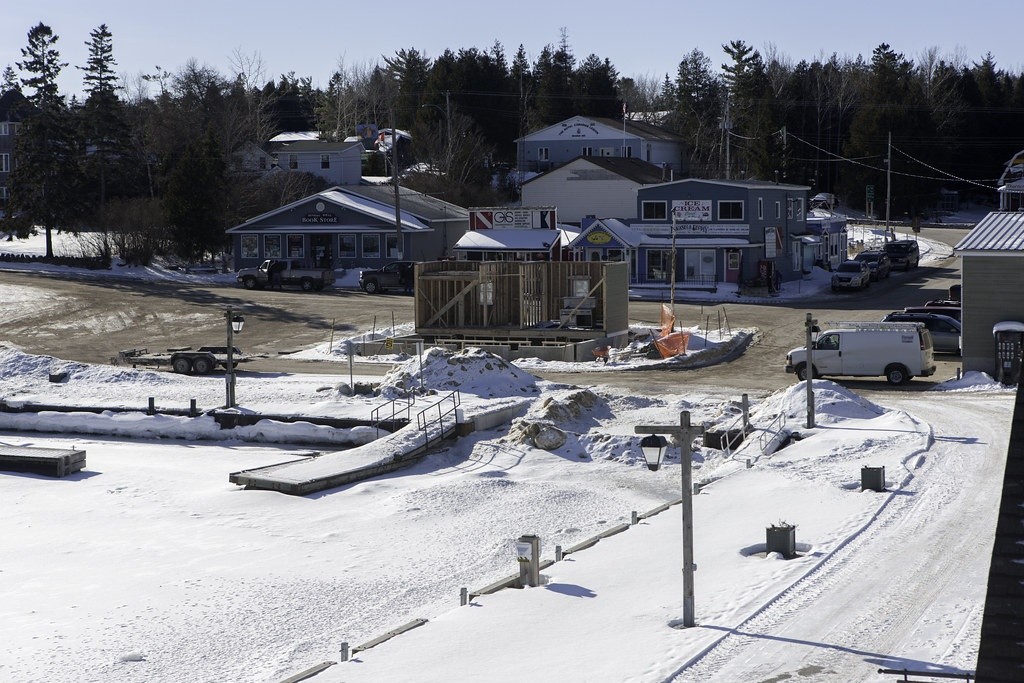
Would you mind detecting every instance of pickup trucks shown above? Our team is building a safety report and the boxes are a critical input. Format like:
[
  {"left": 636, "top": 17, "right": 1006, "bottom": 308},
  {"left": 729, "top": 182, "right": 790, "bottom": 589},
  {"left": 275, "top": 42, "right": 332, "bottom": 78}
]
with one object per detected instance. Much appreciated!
[{"left": 237, "top": 258, "right": 336, "bottom": 292}]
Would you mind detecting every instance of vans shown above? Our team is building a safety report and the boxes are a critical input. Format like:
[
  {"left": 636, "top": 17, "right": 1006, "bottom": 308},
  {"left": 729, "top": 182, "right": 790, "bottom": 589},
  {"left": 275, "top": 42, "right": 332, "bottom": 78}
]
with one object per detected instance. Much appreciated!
[{"left": 784, "top": 319, "right": 936, "bottom": 385}]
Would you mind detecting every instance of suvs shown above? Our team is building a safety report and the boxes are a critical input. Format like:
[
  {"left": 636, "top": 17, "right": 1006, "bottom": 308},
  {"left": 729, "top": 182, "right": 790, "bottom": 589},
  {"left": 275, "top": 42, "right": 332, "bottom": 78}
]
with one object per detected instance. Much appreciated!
[
  {"left": 855, "top": 250, "right": 891, "bottom": 281},
  {"left": 832, "top": 258, "right": 870, "bottom": 290},
  {"left": 881, "top": 311, "right": 962, "bottom": 357}
]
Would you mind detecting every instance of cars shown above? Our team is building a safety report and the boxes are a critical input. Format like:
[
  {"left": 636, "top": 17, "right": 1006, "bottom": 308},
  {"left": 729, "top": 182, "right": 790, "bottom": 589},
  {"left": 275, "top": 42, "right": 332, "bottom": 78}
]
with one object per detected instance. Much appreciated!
[
  {"left": 359, "top": 261, "right": 416, "bottom": 294},
  {"left": 883, "top": 239, "right": 920, "bottom": 271},
  {"left": 904, "top": 306, "right": 961, "bottom": 321},
  {"left": 924, "top": 300, "right": 961, "bottom": 306}
]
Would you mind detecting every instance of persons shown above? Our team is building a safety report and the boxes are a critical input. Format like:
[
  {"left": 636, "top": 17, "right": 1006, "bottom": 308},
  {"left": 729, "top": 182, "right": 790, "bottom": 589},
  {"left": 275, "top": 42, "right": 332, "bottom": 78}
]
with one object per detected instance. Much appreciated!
[
  {"left": 266, "top": 259, "right": 284, "bottom": 292},
  {"left": 403, "top": 262, "right": 414, "bottom": 296}
]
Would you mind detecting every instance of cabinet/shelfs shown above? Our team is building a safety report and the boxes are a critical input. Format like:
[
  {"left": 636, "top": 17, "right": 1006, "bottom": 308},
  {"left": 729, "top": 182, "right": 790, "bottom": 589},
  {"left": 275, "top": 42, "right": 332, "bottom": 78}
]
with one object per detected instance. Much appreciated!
[
  {"left": 564, "top": 297, "right": 597, "bottom": 308},
  {"left": 560, "top": 309, "right": 596, "bottom": 328}
]
